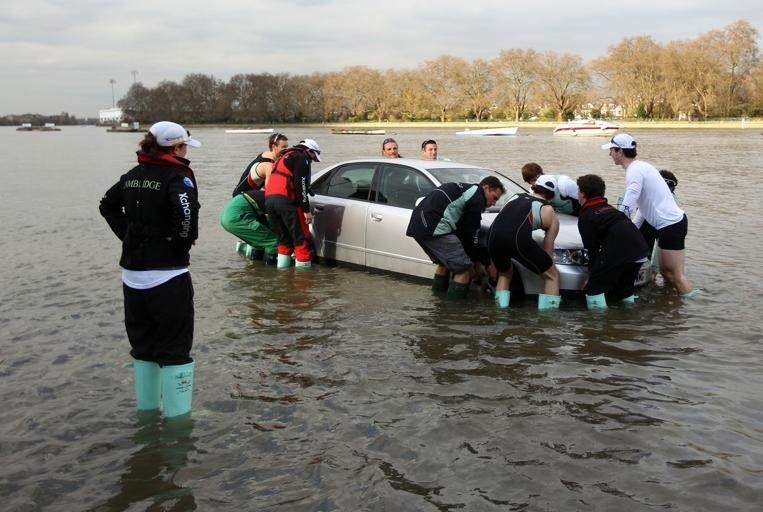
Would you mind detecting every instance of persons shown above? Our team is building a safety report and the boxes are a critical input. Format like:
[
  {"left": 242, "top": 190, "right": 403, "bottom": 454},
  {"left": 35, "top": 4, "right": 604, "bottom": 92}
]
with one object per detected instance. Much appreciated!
[
  {"left": 405, "top": 176, "right": 505, "bottom": 299},
  {"left": 221, "top": 190, "right": 279, "bottom": 267},
  {"left": 422, "top": 138, "right": 438, "bottom": 162},
  {"left": 264, "top": 139, "right": 325, "bottom": 270},
  {"left": 487, "top": 176, "right": 562, "bottom": 311},
  {"left": 232, "top": 132, "right": 288, "bottom": 259},
  {"left": 602, "top": 133, "right": 692, "bottom": 296},
  {"left": 382, "top": 140, "right": 401, "bottom": 159},
  {"left": 99, "top": 121, "right": 201, "bottom": 417},
  {"left": 577, "top": 175, "right": 650, "bottom": 310},
  {"left": 618, "top": 169, "right": 678, "bottom": 283},
  {"left": 521, "top": 163, "right": 580, "bottom": 217}
]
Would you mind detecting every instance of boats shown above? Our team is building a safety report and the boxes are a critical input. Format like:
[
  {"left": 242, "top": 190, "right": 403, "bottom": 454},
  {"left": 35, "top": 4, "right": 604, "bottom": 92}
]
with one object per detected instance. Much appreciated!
[
  {"left": 456, "top": 125, "right": 519, "bottom": 136},
  {"left": 223, "top": 127, "right": 277, "bottom": 134},
  {"left": 16, "top": 121, "right": 62, "bottom": 131},
  {"left": 330, "top": 127, "right": 388, "bottom": 136},
  {"left": 553, "top": 120, "right": 619, "bottom": 137},
  {"left": 105, "top": 122, "right": 149, "bottom": 132}
]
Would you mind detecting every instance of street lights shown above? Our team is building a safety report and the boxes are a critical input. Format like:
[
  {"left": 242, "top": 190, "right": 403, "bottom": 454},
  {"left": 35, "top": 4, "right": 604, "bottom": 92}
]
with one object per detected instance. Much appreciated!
[
  {"left": 131, "top": 70, "right": 139, "bottom": 82},
  {"left": 110, "top": 78, "right": 116, "bottom": 107}
]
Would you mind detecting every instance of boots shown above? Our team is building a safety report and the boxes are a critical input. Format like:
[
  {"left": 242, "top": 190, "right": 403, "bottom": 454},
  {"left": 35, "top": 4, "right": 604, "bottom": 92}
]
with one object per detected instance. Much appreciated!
[
  {"left": 622, "top": 295, "right": 634, "bottom": 304},
  {"left": 586, "top": 293, "right": 607, "bottom": 309},
  {"left": 133, "top": 359, "right": 161, "bottom": 412},
  {"left": 277, "top": 253, "right": 291, "bottom": 269},
  {"left": 161, "top": 358, "right": 195, "bottom": 418},
  {"left": 494, "top": 290, "right": 512, "bottom": 309},
  {"left": 295, "top": 258, "right": 312, "bottom": 268},
  {"left": 432, "top": 274, "right": 449, "bottom": 295},
  {"left": 537, "top": 293, "right": 561, "bottom": 310},
  {"left": 448, "top": 280, "right": 468, "bottom": 300},
  {"left": 236, "top": 241, "right": 277, "bottom": 267}
]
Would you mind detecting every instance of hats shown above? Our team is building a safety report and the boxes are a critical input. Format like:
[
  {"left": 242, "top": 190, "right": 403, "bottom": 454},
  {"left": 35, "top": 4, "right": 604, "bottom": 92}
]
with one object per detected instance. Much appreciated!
[
  {"left": 601, "top": 133, "right": 637, "bottom": 149},
  {"left": 148, "top": 120, "right": 201, "bottom": 148},
  {"left": 535, "top": 174, "right": 558, "bottom": 192},
  {"left": 299, "top": 138, "right": 321, "bottom": 162}
]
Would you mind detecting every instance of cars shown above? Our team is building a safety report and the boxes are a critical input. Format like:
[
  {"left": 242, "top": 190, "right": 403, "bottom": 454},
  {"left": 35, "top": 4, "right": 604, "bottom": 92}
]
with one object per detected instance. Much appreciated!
[{"left": 307, "top": 157, "right": 656, "bottom": 307}]
